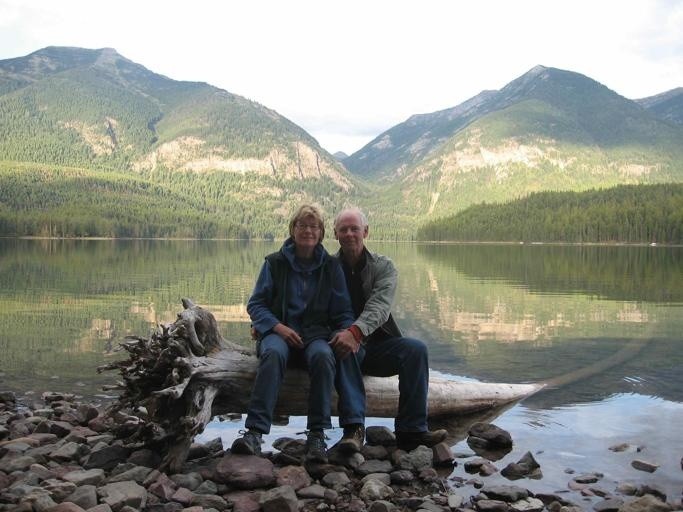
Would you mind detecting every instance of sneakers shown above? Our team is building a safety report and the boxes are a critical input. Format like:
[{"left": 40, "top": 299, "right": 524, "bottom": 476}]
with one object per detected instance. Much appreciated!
[
  {"left": 303, "top": 431, "right": 329, "bottom": 463},
  {"left": 338, "top": 422, "right": 365, "bottom": 452},
  {"left": 393, "top": 426, "right": 449, "bottom": 448},
  {"left": 231, "top": 429, "right": 262, "bottom": 455}
]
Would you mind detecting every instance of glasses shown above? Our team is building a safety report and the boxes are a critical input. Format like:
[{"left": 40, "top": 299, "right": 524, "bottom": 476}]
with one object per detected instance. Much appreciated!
[{"left": 294, "top": 222, "right": 320, "bottom": 230}]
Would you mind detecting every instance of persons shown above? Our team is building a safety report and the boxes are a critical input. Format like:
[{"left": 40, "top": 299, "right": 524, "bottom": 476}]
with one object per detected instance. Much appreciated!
[
  {"left": 227, "top": 204, "right": 361, "bottom": 464},
  {"left": 249, "top": 207, "right": 449, "bottom": 452}
]
